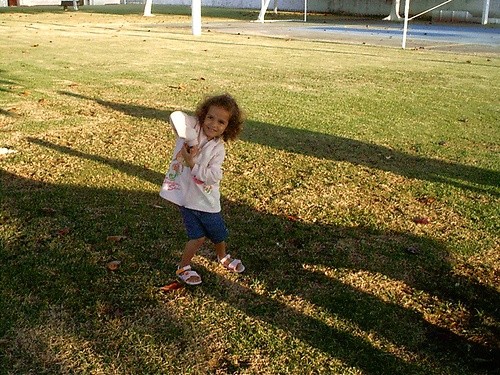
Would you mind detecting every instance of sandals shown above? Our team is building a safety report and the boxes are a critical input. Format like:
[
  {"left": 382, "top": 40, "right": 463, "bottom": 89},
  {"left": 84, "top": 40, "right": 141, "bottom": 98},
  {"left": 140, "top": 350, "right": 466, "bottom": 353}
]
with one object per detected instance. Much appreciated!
[
  {"left": 176, "top": 264, "right": 202, "bottom": 285},
  {"left": 217, "top": 254, "right": 245, "bottom": 272}
]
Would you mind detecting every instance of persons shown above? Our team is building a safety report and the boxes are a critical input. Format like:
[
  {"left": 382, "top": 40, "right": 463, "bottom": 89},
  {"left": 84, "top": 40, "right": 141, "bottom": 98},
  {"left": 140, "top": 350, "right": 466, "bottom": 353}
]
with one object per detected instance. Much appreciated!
[{"left": 159, "top": 93, "right": 245, "bottom": 285}]
